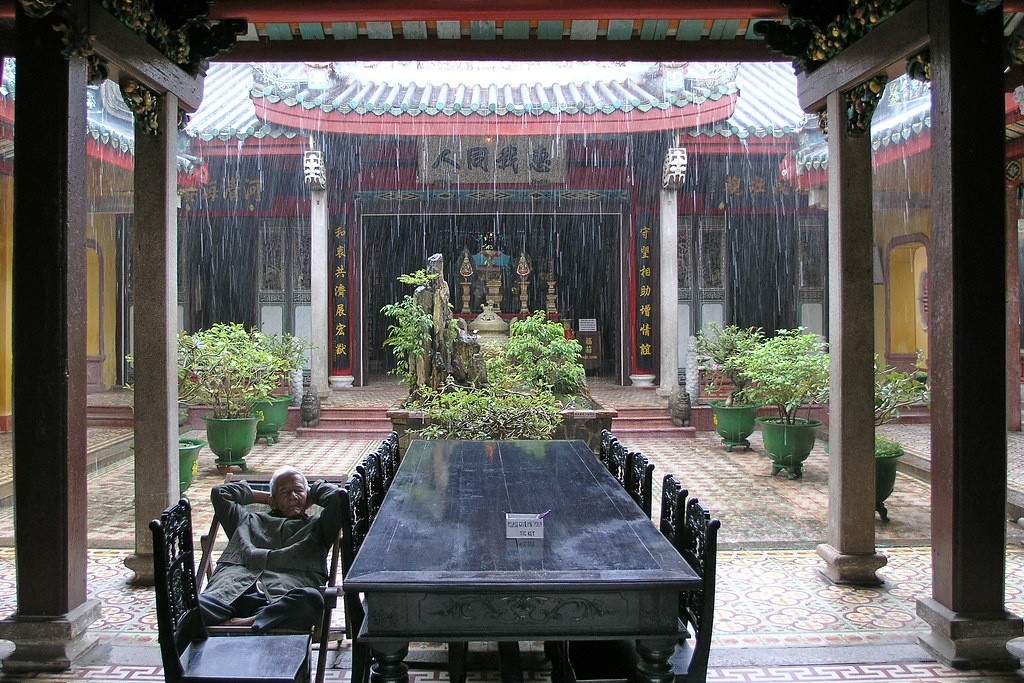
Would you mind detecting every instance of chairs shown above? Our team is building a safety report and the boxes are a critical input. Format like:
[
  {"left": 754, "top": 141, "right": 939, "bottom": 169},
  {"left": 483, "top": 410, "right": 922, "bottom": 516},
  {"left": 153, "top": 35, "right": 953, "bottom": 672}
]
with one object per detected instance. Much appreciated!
[{"left": 148, "top": 431, "right": 722, "bottom": 683}]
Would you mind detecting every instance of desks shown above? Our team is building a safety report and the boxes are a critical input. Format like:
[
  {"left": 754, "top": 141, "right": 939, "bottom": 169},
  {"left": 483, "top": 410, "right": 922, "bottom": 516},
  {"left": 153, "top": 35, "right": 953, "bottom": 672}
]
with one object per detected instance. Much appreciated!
[{"left": 342, "top": 437, "right": 704, "bottom": 683}]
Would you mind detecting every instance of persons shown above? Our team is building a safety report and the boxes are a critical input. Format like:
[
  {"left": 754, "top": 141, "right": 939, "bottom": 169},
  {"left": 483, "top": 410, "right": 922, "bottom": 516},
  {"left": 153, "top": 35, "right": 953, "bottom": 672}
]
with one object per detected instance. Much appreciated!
[{"left": 173, "top": 466, "right": 342, "bottom": 656}]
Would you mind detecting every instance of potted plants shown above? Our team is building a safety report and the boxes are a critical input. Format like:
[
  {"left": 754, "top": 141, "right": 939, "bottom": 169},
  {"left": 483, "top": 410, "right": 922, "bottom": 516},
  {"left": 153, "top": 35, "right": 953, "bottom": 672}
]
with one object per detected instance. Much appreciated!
[
  {"left": 688, "top": 323, "right": 931, "bottom": 526},
  {"left": 123, "top": 322, "right": 320, "bottom": 503}
]
